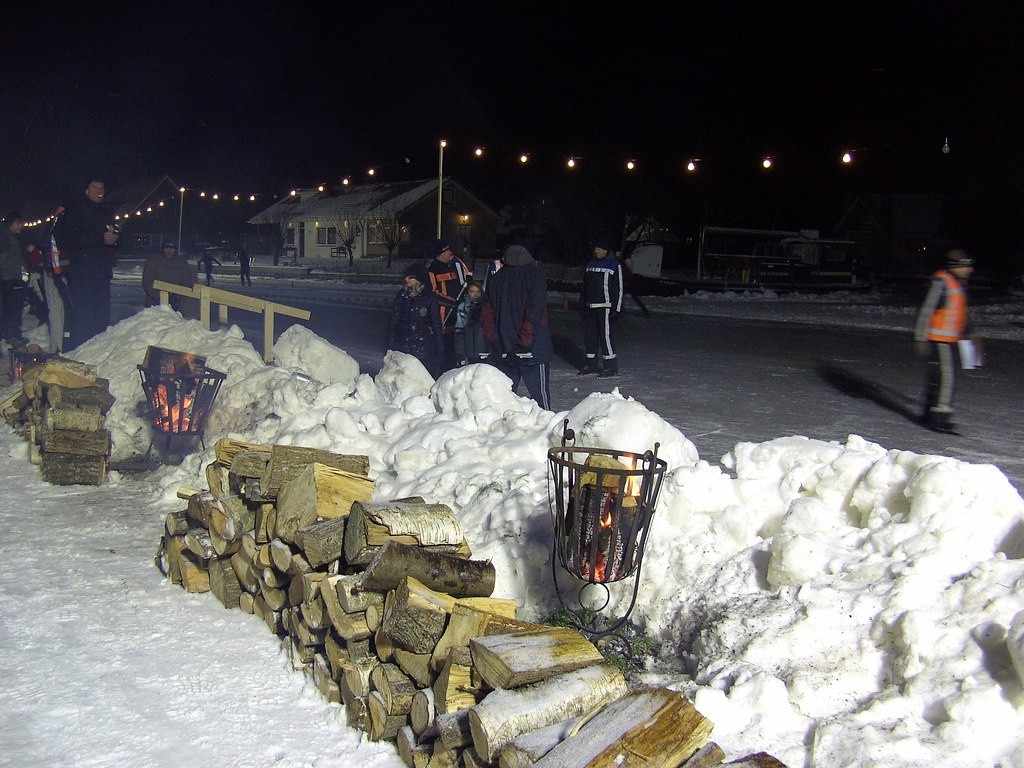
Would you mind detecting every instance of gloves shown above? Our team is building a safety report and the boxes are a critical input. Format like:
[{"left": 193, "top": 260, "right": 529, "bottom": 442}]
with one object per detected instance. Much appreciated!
[
  {"left": 505, "top": 348, "right": 526, "bottom": 363},
  {"left": 608, "top": 309, "right": 619, "bottom": 323},
  {"left": 915, "top": 340, "right": 931, "bottom": 358},
  {"left": 577, "top": 307, "right": 586, "bottom": 319},
  {"left": 465, "top": 274, "right": 474, "bottom": 284},
  {"left": 488, "top": 344, "right": 507, "bottom": 362},
  {"left": 967, "top": 334, "right": 983, "bottom": 351}
]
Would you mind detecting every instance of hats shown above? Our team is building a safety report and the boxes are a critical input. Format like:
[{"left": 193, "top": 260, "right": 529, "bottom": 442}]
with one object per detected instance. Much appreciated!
[
  {"left": 402, "top": 263, "right": 428, "bottom": 283},
  {"left": 27, "top": 246, "right": 44, "bottom": 265},
  {"left": 594, "top": 237, "right": 608, "bottom": 251},
  {"left": 162, "top": 237, "right": 178, "bottom": 248},
  {"left": 947, "top": 249, "right": 973, "bottom": 268},
  {"left": 433, "top": 239, "right": 451, "bottom": 258}
]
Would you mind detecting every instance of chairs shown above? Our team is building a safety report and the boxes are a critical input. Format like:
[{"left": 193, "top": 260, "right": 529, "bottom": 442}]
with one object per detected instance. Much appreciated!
[{"left": 331, "top": 247, "right": 348, "bottom": 259}]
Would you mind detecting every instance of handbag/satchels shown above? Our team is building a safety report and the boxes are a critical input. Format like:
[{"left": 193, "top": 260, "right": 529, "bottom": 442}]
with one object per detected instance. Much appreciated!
[{"left": 168, "top": 295, "right": 182, "bottom": 310}]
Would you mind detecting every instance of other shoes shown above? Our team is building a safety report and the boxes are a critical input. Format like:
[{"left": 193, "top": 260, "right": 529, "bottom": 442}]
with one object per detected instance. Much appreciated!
[
  {"left": 15, "top": 334, "right": 29, "bottom": 344},
  {"left": 6, "top": 336, "right": 24, "bottom": 348}
]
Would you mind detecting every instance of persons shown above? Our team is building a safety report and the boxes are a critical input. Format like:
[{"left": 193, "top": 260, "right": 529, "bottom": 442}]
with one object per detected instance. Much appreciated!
[
  {"left": 142, "top": 239, "right": 195, "bottom": 312},
  {"left": 0, "top": 172, "right": 119, "bottom": 353},
  {"left": 914, "top": 249, "right": 977, "bottom": 431},
  {"left": 577, "top": 239, "right": 625, "bottom": 379},
  {"left": 234, "top": 249, "right": 252, "bottom": 287},
  {"left": 198, "top": 252, "right": 221, "bottom": 286},
  {"left": 387, "top": 237, "right": 551, "bottom": 412}
]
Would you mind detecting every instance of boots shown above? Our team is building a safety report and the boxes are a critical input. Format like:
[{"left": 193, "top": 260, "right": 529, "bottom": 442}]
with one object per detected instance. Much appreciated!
[
  {"left": 599, "top": 354, "right": 619, "bottom": 378},
  {"left": 930, "top": 406, "right": 961, "bottom": 434},
  {"left": 577, "top": 355, "right": 602, "bottom": 376}
]
